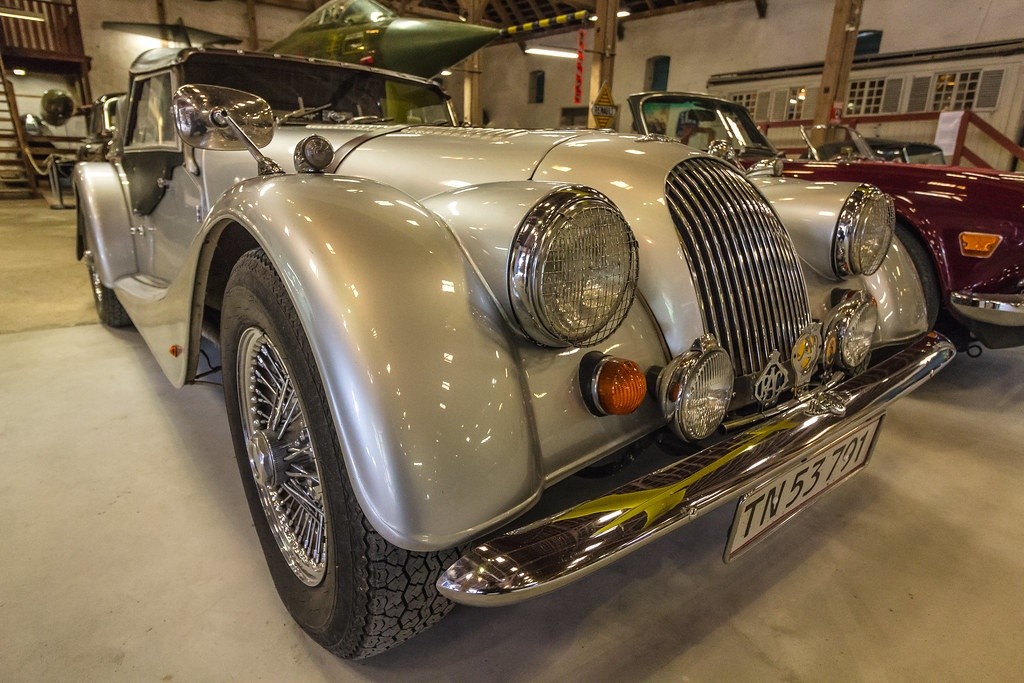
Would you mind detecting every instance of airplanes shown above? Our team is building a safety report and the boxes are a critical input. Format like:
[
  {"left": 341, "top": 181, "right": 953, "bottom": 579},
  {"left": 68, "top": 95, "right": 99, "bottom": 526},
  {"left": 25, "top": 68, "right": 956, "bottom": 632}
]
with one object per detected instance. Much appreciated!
[{"left": 102, "top": 0, "right": 590, "bottom": 82}]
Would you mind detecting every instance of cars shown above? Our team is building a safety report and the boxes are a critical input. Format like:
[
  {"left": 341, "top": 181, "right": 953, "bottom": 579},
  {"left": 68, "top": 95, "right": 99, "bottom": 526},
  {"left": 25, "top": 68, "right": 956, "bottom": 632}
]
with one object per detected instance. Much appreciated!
[
  {"left": 626, "top": 92, "right": 1023, "bottom": 333},
  {"left": 78, "top": 91, "right": 126, "bottom": 162},
  {"left": 73, "top": 43, "right": 960, "bottom": 663}
]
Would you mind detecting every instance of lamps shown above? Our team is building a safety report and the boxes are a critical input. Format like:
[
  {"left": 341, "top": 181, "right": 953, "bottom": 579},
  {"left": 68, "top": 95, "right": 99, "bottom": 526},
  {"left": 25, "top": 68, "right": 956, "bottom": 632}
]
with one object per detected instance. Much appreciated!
[{"left": 0, "top": 8, "right": 45, "bottom": 22}]
